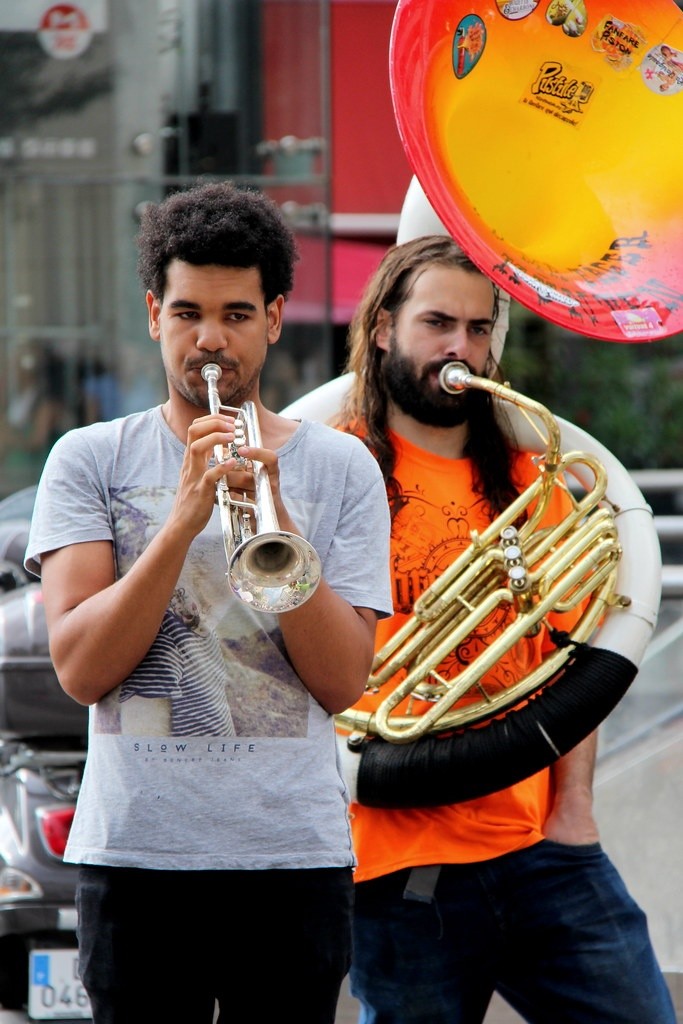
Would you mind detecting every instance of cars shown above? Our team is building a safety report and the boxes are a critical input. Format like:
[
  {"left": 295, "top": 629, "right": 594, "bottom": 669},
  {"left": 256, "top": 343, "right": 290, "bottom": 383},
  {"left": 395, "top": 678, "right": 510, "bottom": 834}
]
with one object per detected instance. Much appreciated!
[{"left": 1, "top": 484, "right": 91, "bottom": 1010}]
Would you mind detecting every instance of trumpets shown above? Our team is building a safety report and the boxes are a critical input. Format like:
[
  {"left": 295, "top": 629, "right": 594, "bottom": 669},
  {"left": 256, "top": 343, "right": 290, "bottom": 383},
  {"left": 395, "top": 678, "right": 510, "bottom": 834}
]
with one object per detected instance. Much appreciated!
[{"left": 199, "top": 362, "right": 321, "bottom": 616}]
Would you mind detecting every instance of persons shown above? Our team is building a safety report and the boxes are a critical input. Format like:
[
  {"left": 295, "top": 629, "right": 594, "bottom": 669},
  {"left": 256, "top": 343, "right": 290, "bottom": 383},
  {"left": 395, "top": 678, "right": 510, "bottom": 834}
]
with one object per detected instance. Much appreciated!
[
  {"left": 326, "top": 235, "right": 679, "bottom": 1023},
  {"left": 1, "top": 332, "right": 114, "bottom": 450},
  {"left": 23, "top": 183, "right": 395, "bottom": 1023}
]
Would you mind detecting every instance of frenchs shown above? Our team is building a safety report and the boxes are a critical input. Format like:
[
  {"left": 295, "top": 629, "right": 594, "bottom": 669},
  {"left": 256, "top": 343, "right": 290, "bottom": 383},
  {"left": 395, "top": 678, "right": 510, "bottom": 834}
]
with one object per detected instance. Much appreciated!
[{"left": 271, "top": 0, "right": 682, "bottom": 808}]
